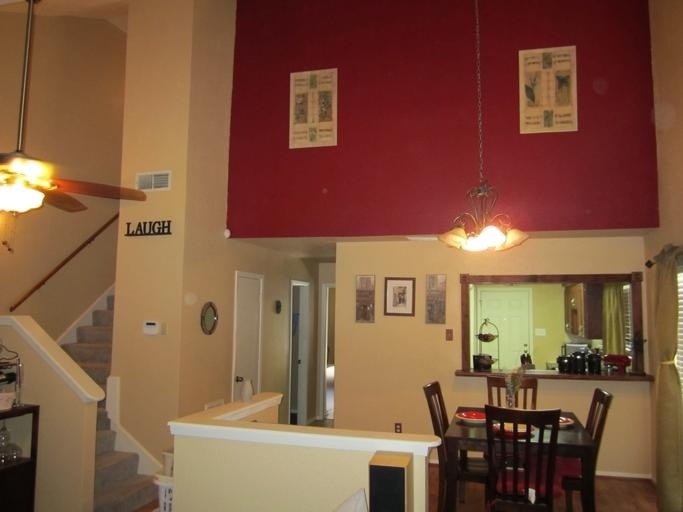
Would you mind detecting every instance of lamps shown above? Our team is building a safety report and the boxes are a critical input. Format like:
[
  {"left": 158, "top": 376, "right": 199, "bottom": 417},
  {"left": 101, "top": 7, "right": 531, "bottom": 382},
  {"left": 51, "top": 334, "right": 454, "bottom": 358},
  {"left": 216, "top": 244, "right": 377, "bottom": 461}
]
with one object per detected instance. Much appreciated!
[
  {"left": 0, "top": 175, "right": 48, "bottom": 215},
  {"left": 437, "top": 1, "right": 532, "bottom": 255}
]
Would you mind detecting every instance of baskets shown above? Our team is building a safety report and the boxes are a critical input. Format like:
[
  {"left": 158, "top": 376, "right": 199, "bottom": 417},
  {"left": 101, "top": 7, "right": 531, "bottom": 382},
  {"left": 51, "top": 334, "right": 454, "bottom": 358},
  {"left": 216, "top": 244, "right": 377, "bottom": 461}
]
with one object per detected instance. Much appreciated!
[{"left": 152, "top": 469, "right": 173, "bottom": 512}]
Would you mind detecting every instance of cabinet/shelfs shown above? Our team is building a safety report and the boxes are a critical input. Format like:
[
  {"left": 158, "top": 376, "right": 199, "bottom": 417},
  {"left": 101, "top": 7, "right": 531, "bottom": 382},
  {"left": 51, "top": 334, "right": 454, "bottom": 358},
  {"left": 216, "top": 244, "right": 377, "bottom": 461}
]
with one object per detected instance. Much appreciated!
[
  {"left": 0, "top": 403, "right": 40, "bottom": 512},
  {"left": 564, "top": 282, "right": 602, "bottom": 339}
]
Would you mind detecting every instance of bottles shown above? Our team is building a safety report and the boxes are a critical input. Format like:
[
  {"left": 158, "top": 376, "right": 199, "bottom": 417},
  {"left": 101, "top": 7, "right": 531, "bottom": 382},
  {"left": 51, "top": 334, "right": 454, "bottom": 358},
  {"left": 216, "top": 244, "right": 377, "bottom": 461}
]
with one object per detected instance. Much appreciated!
[
  {"left": 240, "top": 379, "right": 253, "bottom": 402},
  {"left": 520, "top": 343, "right": 533, "bottom": 366}
]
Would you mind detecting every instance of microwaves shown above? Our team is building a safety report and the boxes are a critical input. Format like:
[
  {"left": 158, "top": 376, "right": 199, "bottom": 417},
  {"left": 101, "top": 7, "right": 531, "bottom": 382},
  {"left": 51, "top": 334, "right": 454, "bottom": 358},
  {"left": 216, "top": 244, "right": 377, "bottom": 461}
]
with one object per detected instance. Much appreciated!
[{"left": 557, "top": 352, "right": 602, "bottom": 375}]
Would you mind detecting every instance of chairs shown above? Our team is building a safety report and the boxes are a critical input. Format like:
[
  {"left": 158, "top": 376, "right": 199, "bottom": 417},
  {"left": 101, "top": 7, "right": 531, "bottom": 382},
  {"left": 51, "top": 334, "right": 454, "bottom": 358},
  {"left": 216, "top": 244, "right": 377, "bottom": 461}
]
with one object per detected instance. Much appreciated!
[
  {"left": 485, "top": 374, "right": 538, "bottom": 410},
  {"left": 481, "top": 404, "right": 561, "bottom": 512},
  {"left": 561, "top": 387, "right": 613, "bottom": 511},
  {"left": 422, "top": 380, "right": 498, "bottom": 512}
]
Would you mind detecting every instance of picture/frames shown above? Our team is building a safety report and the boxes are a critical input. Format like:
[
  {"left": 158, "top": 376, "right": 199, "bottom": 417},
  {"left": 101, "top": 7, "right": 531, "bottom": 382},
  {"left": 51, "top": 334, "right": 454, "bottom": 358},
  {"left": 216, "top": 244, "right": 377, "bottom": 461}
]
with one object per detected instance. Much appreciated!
[{"left": 383, "top": 276, "right": 416, "bottom": 316}]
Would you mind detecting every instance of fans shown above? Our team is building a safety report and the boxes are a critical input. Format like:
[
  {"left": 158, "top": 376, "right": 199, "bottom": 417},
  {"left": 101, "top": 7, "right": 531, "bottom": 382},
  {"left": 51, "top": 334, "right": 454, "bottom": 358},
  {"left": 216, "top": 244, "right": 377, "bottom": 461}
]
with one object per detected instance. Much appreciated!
[{"left": 0, "top": 1, "right": 149, "bottom": 215}]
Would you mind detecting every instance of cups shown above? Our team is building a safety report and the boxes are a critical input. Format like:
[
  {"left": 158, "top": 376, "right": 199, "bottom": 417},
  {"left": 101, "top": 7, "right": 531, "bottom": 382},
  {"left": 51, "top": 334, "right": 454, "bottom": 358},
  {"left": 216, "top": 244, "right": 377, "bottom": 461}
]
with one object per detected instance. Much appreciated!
[
  {"left": 0, "top": 422, "right": 21, "bottom": 466},
  {"left": 472, "top": 355, "right": 492, "bottom": 371}
]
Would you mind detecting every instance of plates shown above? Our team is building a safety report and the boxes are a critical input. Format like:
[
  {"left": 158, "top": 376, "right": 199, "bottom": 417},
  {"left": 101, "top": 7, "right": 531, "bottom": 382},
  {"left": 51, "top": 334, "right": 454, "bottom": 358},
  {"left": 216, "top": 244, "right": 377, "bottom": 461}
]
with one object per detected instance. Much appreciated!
[
  {"left": 493, "top": 422, "right": 536, "bottom": 436},
  {"left": 547, "top": 417, "right": 575, "bottom": 429},
  {"left": 455, "top": 413, "right": 488, "bottom": 424}
]
